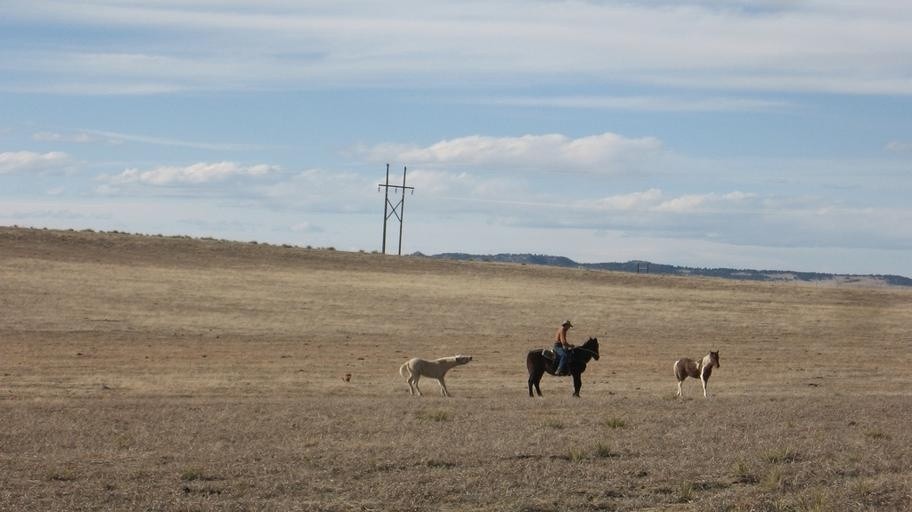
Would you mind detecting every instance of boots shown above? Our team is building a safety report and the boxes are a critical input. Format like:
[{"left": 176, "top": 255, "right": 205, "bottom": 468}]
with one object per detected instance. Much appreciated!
[{"left": 555, "top": 366, "right": 565, "bottom": 376}]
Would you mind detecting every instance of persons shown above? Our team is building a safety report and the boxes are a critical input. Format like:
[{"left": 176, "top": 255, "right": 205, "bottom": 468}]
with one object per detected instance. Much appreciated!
[{"left": 552, "top": 319, "right": 575, "bottom": 374}]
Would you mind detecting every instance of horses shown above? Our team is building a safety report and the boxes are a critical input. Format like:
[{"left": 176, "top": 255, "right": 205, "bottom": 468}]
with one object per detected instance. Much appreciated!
[
  {"left": 673, "top": 349, "right": 721, "bottom": 398},
  {"left": 526, "top": 336, "right": 600, "bottom": 398},
  {"left": 399, "top": 355, "right": 473, "bottom": 397}
]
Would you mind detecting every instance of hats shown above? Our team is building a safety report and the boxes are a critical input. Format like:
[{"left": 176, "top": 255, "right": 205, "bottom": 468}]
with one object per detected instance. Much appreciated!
[{"left": 560, "top": 320, "right": 573, "bottom": 327}]
